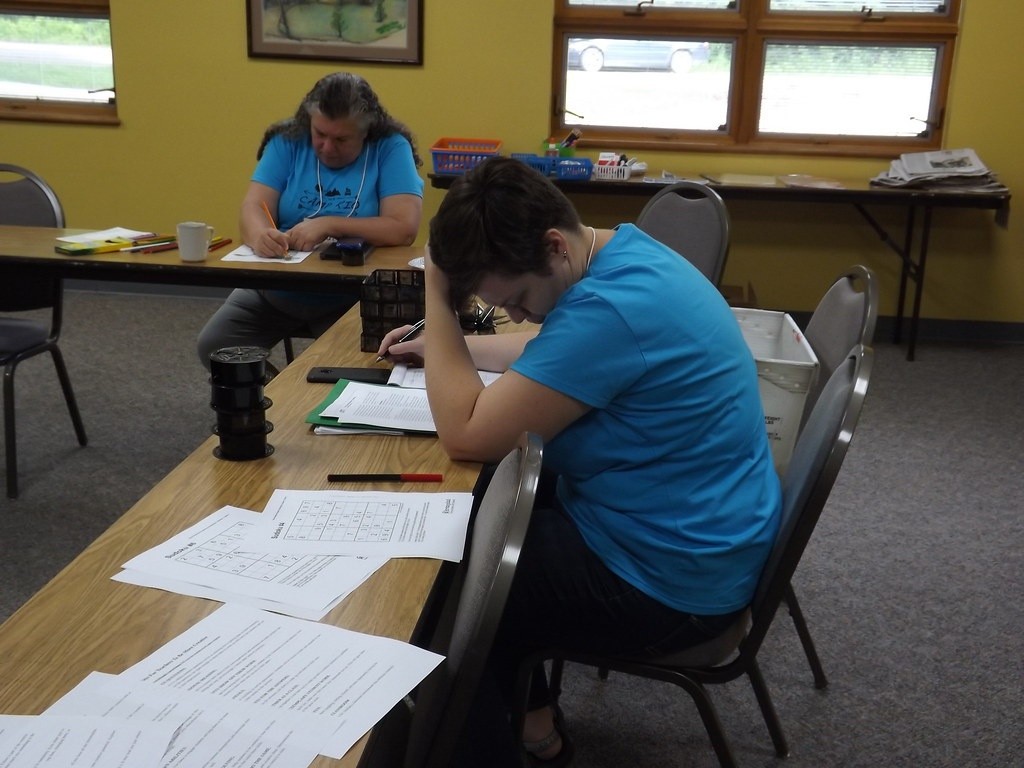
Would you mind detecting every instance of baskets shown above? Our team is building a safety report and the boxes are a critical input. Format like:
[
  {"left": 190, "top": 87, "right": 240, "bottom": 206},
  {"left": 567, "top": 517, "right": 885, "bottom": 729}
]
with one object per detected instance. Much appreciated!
[
  {"left": 554, "top": 158, "right": 594, "bottom": 180},
  {"left": 594, "top": 163, "right": 631, "bottom": 180},
  {"left": 511, "top": 153, "right": 552, "bottom": 177},
  {"left": 428, "top": 137, "right": 501, "bottom": 173}
]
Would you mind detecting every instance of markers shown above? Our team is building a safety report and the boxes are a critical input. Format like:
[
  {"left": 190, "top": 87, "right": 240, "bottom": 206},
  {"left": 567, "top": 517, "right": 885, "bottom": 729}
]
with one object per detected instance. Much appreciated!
[{"left": 620, "top": 158, "right": 637, "bottom": 172}]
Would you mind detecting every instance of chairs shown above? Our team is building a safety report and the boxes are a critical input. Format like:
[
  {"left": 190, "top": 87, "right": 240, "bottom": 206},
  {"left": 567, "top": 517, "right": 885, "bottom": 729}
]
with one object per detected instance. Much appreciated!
[
  {"left": 0, "top": 164, "right": 90, "bottom": 498},
  {"left": 784, "top": 264, "right": 879, "bottom": 692},
  {"left": 637, "top": 179, "right": 733, "bottom": 286},
  {"left": 549, "top": 344, "right": 875, "bottom": 768},
  {"left": 401, "top": 431, "right": 546, "bottom": 768}
]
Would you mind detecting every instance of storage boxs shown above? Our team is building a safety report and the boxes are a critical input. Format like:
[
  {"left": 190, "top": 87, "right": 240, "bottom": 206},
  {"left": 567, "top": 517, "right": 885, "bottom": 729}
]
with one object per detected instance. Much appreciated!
[{"left": 730, "top": 307, "right": 822, "bottom": 488}]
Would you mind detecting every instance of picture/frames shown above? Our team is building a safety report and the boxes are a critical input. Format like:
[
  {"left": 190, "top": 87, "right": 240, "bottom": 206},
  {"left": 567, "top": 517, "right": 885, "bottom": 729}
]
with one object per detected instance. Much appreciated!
[{"left": 246, "top": 0, "right": 426, "bottom": 68}]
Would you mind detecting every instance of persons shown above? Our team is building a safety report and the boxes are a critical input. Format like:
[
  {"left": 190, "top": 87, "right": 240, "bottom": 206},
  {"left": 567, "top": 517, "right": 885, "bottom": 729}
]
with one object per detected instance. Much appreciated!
[
  {"left": 380, "top": 159, "right": 782, "bottom": 768},
  {"left": 197, "top": 73, "right": 424, "bottom": 385}
]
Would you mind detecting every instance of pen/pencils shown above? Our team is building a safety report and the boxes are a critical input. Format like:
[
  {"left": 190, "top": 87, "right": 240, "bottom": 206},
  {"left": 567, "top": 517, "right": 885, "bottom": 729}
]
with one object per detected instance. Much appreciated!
[
  {"left": 375, "top": 319, "right": 425, "bottom": 363},
  {"left": 119, "top": 240, "right": 178, "bottom": 253},
  {"left": 208, "top": 236, "right": 232, "bottom": 252},
  {"left": 327, "top": 472, "right": 443, "bottom": 482},
  {"left": 261, "top": 201, "right": 289, "bottom": 256}
]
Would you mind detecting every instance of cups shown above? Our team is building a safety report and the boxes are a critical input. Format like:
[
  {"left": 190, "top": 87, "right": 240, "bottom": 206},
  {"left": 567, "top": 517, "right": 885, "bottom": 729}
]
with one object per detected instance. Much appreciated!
[{"left": 176, "top": 221, "right": 214, "bottom": 262}]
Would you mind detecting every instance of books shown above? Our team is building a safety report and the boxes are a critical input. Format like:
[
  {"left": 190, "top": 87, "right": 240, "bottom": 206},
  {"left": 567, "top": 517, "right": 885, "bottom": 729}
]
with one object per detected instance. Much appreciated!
[{"left": 304, "top": 361, "right": 504, "bottom": 436}]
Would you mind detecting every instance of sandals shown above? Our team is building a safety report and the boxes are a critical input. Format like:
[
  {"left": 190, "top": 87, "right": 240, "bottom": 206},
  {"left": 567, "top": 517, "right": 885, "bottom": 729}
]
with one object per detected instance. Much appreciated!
[{"left": 522, "top": 721, "right": 575, "bottom": 768}]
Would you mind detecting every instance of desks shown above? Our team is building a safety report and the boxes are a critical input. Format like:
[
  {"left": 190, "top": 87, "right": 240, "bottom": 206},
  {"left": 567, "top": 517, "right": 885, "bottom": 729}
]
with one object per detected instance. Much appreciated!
[
  {"left": 428, "top": 168, "right": 1011, "bottom": 360},
  {"left": 1, "top": 224, "right": 546, "bottom": 767}
]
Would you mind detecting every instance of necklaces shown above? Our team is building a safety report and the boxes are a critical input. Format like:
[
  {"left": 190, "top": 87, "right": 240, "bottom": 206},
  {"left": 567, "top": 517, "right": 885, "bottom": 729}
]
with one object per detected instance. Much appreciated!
[
  {"left": 586, "top": 227, "right": 595, "bottom": 272},
  {"left": 307, "top": 143, "right": 369, "bottom": 217}
]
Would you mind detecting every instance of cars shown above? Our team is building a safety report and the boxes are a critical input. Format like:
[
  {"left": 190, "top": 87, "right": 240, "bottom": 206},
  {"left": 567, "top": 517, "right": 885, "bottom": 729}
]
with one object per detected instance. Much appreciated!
[{"left": 567, "top": 37, "right": 713, "bottom": 75}]
